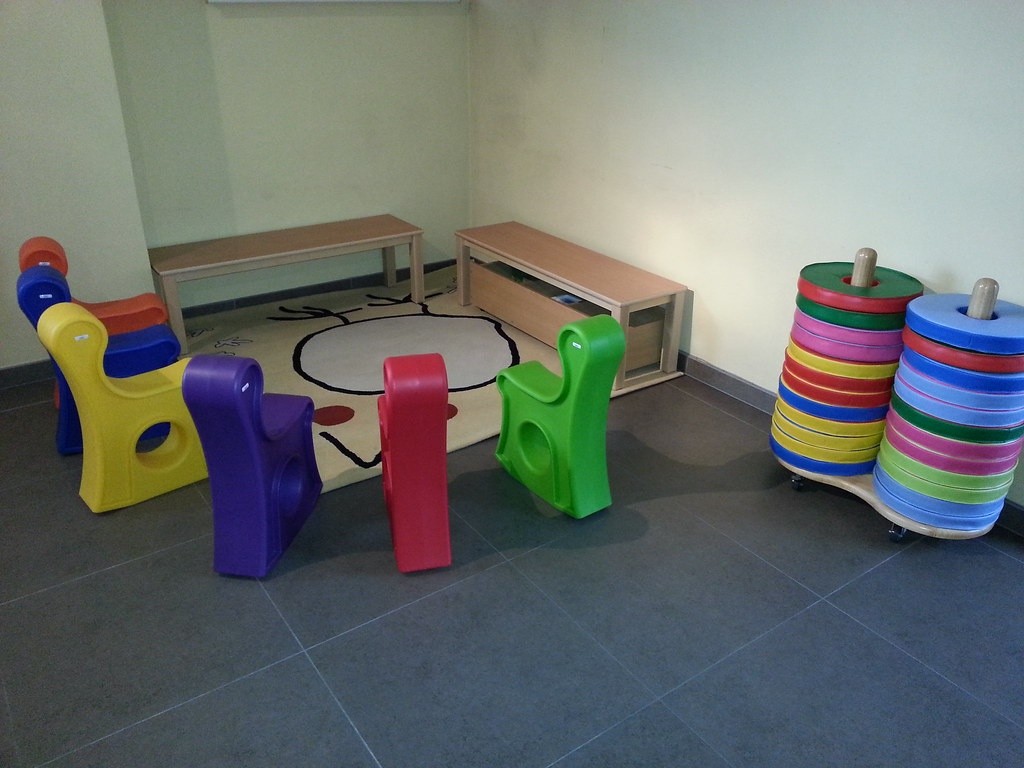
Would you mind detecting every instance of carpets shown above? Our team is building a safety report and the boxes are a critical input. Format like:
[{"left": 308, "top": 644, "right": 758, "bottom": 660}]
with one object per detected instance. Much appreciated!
[{"left": 177, "top": 261, "right": 683, "bottom": 493}]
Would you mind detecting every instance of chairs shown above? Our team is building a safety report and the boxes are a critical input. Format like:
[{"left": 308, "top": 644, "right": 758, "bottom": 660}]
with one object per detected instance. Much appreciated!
[
  {"left": 15, "top": 266, "right": 182, "bottom": 456},
  {"left": 375, "top": 354, "right": 452, "bottom": 574},
  {"left": 496, "top": 315, "right": 626, "bottom": 519},
  {"left": 18, "top": 235, "right": 169, "bottom": 410},
  {"left": 183, "top": 353, "right": 322, "bottom": 579},
  {"left": 36, "top": 302, "right": 209, "bottom": 514}
]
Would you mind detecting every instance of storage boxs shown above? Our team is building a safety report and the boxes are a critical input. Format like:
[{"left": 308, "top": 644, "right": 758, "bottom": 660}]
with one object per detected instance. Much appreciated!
[{"left": 469, "top": 260, "right": 664, "bottom": 373}]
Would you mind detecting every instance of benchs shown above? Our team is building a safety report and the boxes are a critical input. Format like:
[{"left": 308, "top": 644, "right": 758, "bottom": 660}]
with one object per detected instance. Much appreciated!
[
  {"left": 455, "top": 221, "right": 688, "bottom": 391},
  {"left": 147, "top": 214, "right": 425, "bottom": 355}
]
